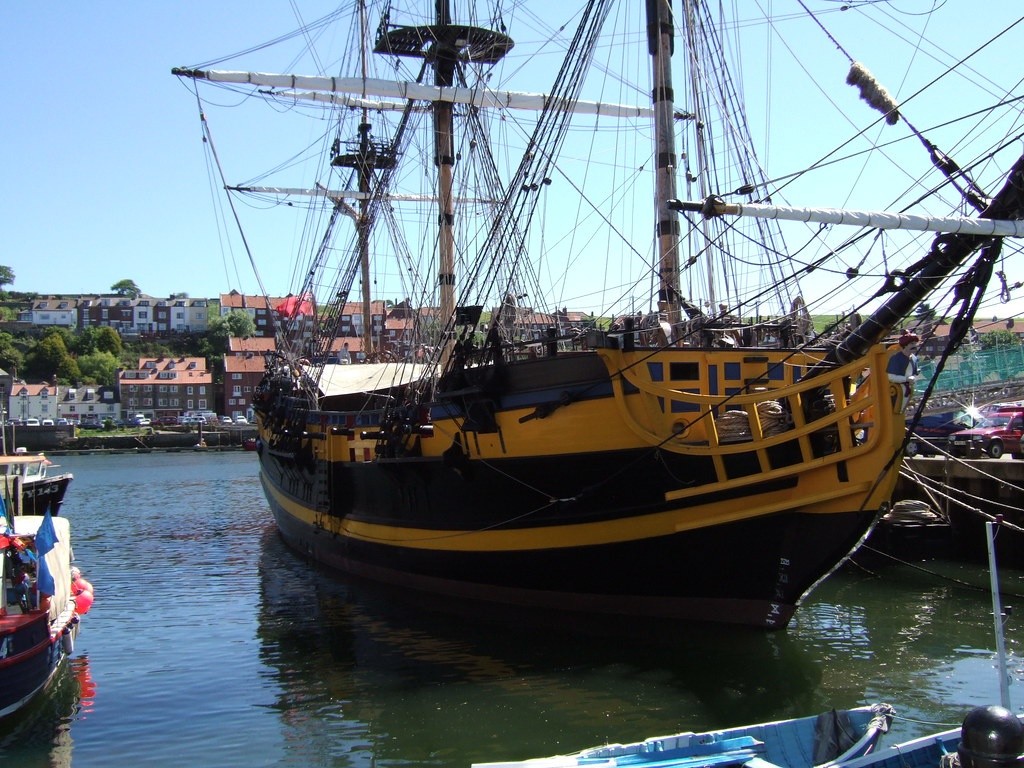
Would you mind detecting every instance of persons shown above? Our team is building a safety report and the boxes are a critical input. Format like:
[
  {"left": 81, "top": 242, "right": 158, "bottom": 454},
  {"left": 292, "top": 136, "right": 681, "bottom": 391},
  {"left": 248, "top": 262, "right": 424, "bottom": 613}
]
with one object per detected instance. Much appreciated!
[
  {"left": 887, "top": 334, "right": 919, "bottom": 383},
  {"left": 338, "top": 342, "right": 351, "bottom": 364}
]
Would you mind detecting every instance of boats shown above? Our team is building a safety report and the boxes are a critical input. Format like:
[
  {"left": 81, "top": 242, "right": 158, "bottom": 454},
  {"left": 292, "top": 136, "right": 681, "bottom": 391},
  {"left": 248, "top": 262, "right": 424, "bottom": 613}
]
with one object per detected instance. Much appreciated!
[
  {"left": 1, "top": 509, "right": 81, "bottom": 722},
  {"left": 0, "top": 404, "right": 75, "bottom": 519},
  {"left": 468, "top": 702, "right": 963, "bottom": 768}
]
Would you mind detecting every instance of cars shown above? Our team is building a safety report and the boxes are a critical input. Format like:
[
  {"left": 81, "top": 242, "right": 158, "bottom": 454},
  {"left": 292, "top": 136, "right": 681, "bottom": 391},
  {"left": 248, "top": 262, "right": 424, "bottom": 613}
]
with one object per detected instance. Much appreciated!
[
  {"left": 235, "top": 415, "right": 249, "bottom": 426},
  {"left": 7, "top": 410, "right": 219, "bottom": 429},
  {"left": 218, "top": 416, "right": 233, "bottom": 425},
  {"left": 903, "top": 397, "right": 1024, "bottom": 460}
]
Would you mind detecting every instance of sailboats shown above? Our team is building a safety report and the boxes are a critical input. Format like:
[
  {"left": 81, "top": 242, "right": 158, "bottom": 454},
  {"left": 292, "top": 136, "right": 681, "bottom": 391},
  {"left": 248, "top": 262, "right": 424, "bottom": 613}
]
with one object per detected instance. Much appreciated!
[{"left": 172, "top": 0, "right": 1024, "bottom": 635}]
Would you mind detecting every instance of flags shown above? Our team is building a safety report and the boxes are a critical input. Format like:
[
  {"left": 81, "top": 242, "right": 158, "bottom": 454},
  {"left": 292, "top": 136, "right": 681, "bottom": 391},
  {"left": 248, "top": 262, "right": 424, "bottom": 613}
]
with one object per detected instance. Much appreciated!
[
  {"left": 37, "top": 511, "right": 58, "bottom": 555},
  {"left": 37, "top": 556, "right": 55, "bottom": 596}
]
